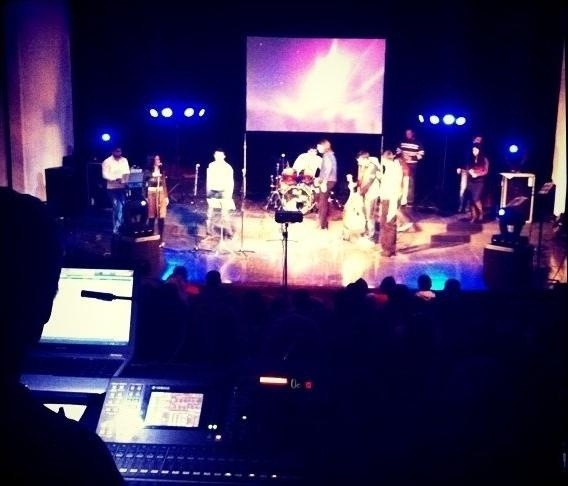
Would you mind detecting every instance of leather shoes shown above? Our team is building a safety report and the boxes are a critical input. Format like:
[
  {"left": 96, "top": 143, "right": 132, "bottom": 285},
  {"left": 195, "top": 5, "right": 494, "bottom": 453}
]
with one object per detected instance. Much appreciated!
[
  {"left": 378, "top": 251, "right": 397, "bottom": 257},
  {"left": 379, "top": 253, "right": 391, "bottom": 258},
  {"left": 159, "top": 241, "right": 166, "bottom": 249}
]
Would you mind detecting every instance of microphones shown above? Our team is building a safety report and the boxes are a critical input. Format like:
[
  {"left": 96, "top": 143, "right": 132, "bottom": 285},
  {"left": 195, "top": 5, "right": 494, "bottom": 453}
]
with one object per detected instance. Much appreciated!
[
  {"left": 81, "top": 290, "right": 114, "bottom": 305},
  {"left": 242, "top": 169, "right": 246, "bottom": 176}
]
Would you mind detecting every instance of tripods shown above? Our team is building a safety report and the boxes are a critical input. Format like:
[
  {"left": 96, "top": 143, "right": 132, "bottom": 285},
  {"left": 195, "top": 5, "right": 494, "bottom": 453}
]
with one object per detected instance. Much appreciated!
[
  {"left": 234, "top": 176, "right": 255, "bottom": 257},
  {"left": 213, "top": 202, "right": 236, "bottom": 255},
  {"left": 263, "top": 163, "right": 285, "bottom": 211}
]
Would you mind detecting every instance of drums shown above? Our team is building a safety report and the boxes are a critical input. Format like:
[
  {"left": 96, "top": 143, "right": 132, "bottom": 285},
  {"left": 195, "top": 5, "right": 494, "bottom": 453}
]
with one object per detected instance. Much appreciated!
[
  {"left": 282, "top": 169, "right": 298, "bottom": 185},
  {"left": 313, "top": 189, "right": 320, "bottom": 210},
  {"left": 300, "top": 176, "right": 314, "bottom": 185}
]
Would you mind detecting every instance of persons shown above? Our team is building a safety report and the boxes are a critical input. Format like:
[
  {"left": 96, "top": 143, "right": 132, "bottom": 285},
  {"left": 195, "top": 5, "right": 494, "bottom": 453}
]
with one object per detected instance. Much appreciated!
[
  {"left": 394, "top": 153, "right": 417, "bottom": 233},
  {"left": 358, "top": 152, "right": 379, "bottom": 238},
  {"left": 102, "top": 144, "right": 131, "bottom": 240},
  {"left": 417, "top": 275, "right": 432, "bottom": 289},
  {"left": 204, "top": 271, "right": 221, "bottom": 281},
  {"left": 444, "top": 278, "right": 461, "bottom": 294},
  {"left": 353, "top": 278, "right": 368, "bottom": 291},
  {"left": 380, "top": 277, "right": 397, "bottom": 292},
  {"left": 141, "top": 153, "right": 169, "bottom": 247},
  {"left": 269, "top": 282, "right": 326, "bottom": 315},
  {"left": 357, "top": 150, "right": 379, "bottom": 180},
  {"left": 317, "top": 138, "right": 337, "bottom": 231},
  {"left": 0, "top": 186, "right": 126, "bottom": 485},
  {"left": 395, "top": 129, "right": 425, "bottom": 202},
  {"left": 139, "top": 280, "right": 190, "bottom": 314},
  {"left": 378, "top": 151, "right": 403, "bottom": 256},
  {"left": 466, "top": 143, "right": 489, "bottom": 223},
  {"left": 211, "top": 287, "right": 267, "bottom": 319},
  {"left": 173, "top": 267, "right": 188, "bottom": 280},
  {"left": 456, "top": 134, "right": 482, "bottom": 213},
  {"left": 206, "top": 149, "right": 234, "bottom": 200},
  {"left": 292, "top": 145, "right": 322, "bottom": 178}
]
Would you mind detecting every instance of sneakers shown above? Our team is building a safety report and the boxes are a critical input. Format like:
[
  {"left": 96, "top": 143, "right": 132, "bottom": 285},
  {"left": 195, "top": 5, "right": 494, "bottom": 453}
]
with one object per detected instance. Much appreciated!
[
  {"left": 320, "top": 228, "right": 328, "bottom": 232},
  {"left": 317, "top": 225, "right": 321, "bottom": 228}
]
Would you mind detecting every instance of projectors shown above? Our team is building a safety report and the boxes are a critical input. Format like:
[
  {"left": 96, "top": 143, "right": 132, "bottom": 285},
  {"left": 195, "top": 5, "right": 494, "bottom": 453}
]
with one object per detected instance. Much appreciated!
[{"left": 275, "top": 210, "right": 303, "bottom": 223}]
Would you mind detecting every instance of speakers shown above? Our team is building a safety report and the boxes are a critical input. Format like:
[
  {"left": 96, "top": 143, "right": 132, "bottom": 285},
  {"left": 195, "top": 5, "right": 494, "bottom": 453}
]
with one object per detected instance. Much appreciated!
[
  {"left": 110, "top": 233, "right": 164, "bottom": 274},
  {"left": 483, "top": 244, "right": 534, "bottom": 290}
]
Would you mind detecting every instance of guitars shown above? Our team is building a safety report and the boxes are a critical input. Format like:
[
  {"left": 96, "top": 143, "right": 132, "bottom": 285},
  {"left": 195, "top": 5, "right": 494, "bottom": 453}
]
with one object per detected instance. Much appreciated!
[{"left": 344, "top": 175, "right": 365, "bottom": 233}]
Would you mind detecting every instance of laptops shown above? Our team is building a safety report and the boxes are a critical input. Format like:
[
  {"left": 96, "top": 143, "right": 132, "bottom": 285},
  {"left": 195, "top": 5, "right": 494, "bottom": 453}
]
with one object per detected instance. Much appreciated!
[{"left": 18, "top": 267, "right": 135, "bottom": 395}]
